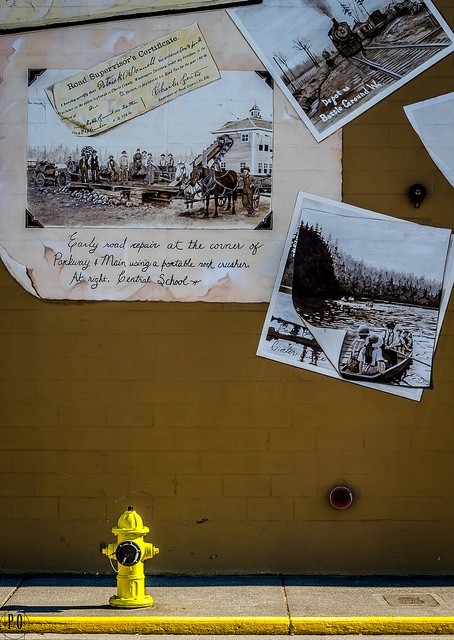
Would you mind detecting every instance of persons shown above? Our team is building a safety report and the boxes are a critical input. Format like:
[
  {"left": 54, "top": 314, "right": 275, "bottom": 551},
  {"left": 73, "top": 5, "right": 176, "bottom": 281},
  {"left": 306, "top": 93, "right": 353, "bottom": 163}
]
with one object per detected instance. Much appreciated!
[
  {"left": 105, "top": 155, "right": 118, "bottom": 180},
  {"left": 119, "top": 151, "right": 130, "bottom": 184},
  {"left": 239, "top": 166, "right": 259, "bottom": 217},
  {"left": 356, "top": 335, "right": 386, "bottom": 375},
  {"left": 157, "top": 154, "right": 166, "bottom": 167},
  {"left": 132, "top": 149, "right": 141, "bottom": 164},
  {"left": 380, "top": 322, "right": 398, "bottom": 350},
  {"left": 142, "top": 151, "right": 147, "bottom": 165},
  {"left": 396, "top": 328, "right": 406, "bottom": 355},
  {"left": 78, "top": 153, "right": 89, "bottom": 183},
  {"left": 167, "top": 153, "right": 175, "bottom": 176},
  {"left": 87, "top": 149, "right": 100, "bottom": 183},
  {"left": 146, "top": 152, "right": 154, "bottom": 187},
  {"left": 348, "top": 326, "right": 369, "bottom": 373},
  {"left": 403, "top": 330, "right": 412, "bottom": 350}
]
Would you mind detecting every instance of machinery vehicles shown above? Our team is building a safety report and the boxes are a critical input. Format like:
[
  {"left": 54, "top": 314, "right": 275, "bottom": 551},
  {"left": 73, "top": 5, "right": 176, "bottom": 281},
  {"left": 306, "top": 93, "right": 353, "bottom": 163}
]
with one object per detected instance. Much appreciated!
[{"left": 190, "top": 134, "right": 234, "bottom": 169}]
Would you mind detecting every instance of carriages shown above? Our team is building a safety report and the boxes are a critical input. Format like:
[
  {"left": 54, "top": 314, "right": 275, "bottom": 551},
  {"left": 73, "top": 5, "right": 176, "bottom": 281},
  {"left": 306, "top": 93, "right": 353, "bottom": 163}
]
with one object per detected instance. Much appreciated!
[{"left": 174, "top": 157, "right": 260, "bottom": 218}]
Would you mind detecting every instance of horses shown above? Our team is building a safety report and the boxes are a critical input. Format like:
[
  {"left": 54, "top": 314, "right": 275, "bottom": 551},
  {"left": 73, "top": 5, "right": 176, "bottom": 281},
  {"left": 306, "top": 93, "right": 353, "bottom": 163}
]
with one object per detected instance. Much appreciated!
[
  {"left": 175, "top": 158, "right": 228, "bottom": 213},
  {"left": 190, "top": 162, "right": 238, "bottom": 218}
]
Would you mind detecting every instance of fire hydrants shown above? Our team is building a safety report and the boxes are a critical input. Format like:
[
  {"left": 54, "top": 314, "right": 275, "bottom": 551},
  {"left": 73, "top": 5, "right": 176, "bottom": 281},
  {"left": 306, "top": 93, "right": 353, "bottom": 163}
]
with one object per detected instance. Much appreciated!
[{"left": 100, "top": 505, "right": 160, "bottom": 609}]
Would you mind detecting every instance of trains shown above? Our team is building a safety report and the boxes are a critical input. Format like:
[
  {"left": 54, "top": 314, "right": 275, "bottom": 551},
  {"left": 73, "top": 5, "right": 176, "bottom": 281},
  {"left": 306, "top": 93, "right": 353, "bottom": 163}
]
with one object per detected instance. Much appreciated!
[
  {"left": 352, "top": 9, "right": 386, "bottom": 39},
  {"left": 327, "top": 17, "right": 363, "bottom": 59}
]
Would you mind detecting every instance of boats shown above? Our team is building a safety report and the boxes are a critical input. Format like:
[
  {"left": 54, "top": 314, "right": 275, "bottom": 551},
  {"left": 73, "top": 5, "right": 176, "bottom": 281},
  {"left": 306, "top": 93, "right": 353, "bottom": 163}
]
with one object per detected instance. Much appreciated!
[{"left": 336, "top": 330, "right": 414, "bottom": 379}]
